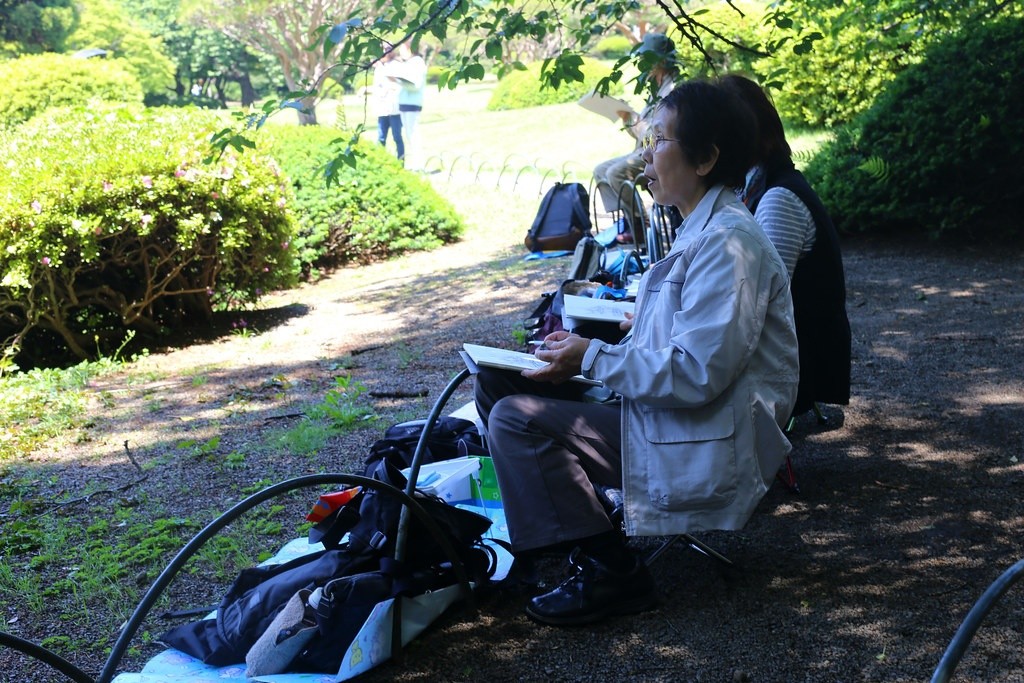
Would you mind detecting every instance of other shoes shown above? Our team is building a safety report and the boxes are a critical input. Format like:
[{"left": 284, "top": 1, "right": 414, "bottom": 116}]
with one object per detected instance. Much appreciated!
[{"left": 617, "top": 227, "right": 643, "bottom": 244}]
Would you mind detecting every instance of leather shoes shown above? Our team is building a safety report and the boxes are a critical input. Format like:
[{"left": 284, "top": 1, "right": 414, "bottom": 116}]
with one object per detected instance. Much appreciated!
[{"left": 525, "top": 553, "right": 663, "bottom": 625}]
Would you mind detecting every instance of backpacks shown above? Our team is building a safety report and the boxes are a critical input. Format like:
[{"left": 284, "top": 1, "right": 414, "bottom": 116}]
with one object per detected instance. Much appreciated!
[
  {"left": 362, "top": 415, "right": 491, "bottom": 466},
  {"left": 525, "top": 182, "right": 591, "bottom": 250},
  {"left": 568, "top": 237, "right": 607, "bottom": 280}
]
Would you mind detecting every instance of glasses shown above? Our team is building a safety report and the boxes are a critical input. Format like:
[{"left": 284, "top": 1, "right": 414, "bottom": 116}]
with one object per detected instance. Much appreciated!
[{"left": 642, "top": 133, "right": 680, "bottom": 152}]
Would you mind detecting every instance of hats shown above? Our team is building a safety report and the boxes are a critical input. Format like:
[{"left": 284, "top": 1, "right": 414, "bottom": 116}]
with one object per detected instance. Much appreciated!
[{"left": 636, "top": 33, "right": 678, "bottom": 59}]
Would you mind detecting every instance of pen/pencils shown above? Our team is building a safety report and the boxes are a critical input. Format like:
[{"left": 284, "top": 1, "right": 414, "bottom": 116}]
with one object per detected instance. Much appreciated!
[
  {"left": 541, "top": 292, "right": 548, "bottom": 297},
  {"left": 528, "top": 341, "right": 557, "bottom": 345},
  {"left": 624, "top": 100, "right": 631, "bottom": 103}
]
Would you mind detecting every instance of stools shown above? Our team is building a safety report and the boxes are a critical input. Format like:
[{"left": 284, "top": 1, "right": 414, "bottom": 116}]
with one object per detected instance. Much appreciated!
[{"left": 569, "top": 402, "right": 829, "bottom": 575}]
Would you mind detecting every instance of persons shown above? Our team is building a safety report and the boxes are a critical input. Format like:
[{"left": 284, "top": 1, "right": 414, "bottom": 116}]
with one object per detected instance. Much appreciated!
[
  {"left": 359, "top": 43, "right": 428, "bottom": 170},
  {"left": 472, "top": 78, "right": 801, "bottom": 624},
  {"left": 593, "top": 0, "right": 854, "bottom": 419}
]
